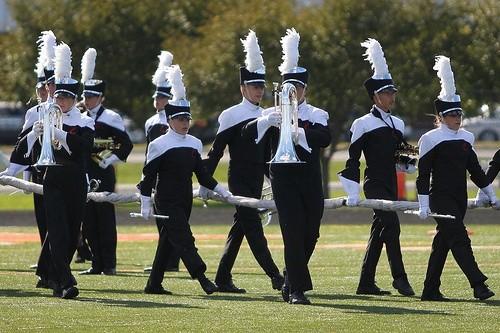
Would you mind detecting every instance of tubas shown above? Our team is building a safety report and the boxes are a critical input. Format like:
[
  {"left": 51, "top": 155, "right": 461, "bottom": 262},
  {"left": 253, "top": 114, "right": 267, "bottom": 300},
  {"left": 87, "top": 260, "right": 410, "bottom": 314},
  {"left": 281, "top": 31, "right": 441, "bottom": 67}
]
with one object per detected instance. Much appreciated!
[
  {"left": 257, "top": 185, "right": 278, "bottom": 227},
  {"left": 31, "top": 97, "right": 64, "bottom": 167},
  {"left": 266, "top": 82, "right": 307, "bottom": 164}
]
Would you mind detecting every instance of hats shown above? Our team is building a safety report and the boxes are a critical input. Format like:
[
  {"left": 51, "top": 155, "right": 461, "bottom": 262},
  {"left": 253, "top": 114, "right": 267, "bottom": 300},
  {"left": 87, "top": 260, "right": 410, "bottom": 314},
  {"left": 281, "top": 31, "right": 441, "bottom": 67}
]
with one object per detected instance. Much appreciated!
[
  {"left": 433, "top": 55, "right": 464, "bottom": 116},
  {"left": 240, "top": 29, "right": 267, "bottom": 88},
  {"left": 278, "top": 27, "right": 308, "bottom": 87},
  {"left": 35, "top": 29, "right": 105, "bottom": 98},
  {"left": 151, "top": 51, "right": 193, "bottom": 120},
  {"left": 360, "top": 38, "right": 398, "bottom": 98}
]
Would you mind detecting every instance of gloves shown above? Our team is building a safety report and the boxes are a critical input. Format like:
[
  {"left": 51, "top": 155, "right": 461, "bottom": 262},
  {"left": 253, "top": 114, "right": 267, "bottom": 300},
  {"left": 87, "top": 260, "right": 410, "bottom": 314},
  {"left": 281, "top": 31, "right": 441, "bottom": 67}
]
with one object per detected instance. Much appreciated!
[
  {"left": 395, "top": 164, "right": 418, "bottom": 173},
  {"left": 0, "top": 120, "right": 120, "bottom": 194},
  {"left": 338, "top": 173, "right": 362, "bottom": 207},
  {"left": 417, "top": 194, "right": 431, "bottom": 220},
  {"left": 140, "top": 195, "right": 152, "bottom": 221},
  {"left": 475, "top": 188, "right": 489, "bottom": 207},
  {"left": 256, "top": 111, "right": 282, "bottom": 144},
  {"left": 214, "top": 183, "right": 233, "bottom": 198},
  {"left": 198, "top": 185, "right": 214, "bottom": 200},
  {"left": 481, "top": 183, "right": 496, "bottom": 204}
]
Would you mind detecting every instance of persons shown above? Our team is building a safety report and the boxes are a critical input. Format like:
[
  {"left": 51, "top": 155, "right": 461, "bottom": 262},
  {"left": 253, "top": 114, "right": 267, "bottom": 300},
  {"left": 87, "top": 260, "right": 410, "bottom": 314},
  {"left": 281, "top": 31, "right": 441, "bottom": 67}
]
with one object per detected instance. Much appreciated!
[
  {"left": 0, "top": 30, "right": 134, "bottom": 299},
  {"left": 242, "top": 28, "right": 332, "bottom": 304},
  {"left": 416, "top": 55, "right": 495, "bottom": 301},
  {"left": 336, "top": 37, "right": 420, "bottom": 296},
  {"left": 143, "top": 50, "right": 180, "bottom": 274},
  {"left": 475, "top": 149, "right": 500, "bottom": 207},
  {"left": 137, "top": 63, "right": 233, "bottom": 296},
  {"left": 198, "top": 28, "right": 284, "bottom": 293}
]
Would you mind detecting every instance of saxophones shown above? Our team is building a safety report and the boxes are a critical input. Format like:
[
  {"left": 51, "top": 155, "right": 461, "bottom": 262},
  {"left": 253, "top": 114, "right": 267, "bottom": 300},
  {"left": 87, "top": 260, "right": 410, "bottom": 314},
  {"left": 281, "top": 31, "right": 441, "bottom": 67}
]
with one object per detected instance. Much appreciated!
[
  {"left": 393, "top": 143, "right": 419, "bottom": 169},
  {"left": 91, "top": 136, "right": 121, "bottom": 164}
]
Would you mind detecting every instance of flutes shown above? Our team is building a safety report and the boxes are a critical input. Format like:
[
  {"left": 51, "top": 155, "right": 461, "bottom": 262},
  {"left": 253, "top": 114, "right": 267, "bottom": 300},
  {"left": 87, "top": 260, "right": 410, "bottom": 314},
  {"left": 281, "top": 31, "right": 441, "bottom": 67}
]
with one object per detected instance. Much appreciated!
[
  {"left": 129, "top": 212, "right": 169, "bottom": 220},
  {"left": 404, "top": 210, "right": 456, "bottom": 219}
]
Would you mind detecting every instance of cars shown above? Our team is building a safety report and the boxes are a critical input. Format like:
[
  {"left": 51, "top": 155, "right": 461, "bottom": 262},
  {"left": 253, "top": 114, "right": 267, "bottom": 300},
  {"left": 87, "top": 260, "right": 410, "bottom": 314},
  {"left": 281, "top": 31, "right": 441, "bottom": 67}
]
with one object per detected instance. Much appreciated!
[
  {"left": 460, "top": 105, "right": 500, "bottom": 143},
  {"left": 0, "top": 101, "right": 27, "bottom": 145}
]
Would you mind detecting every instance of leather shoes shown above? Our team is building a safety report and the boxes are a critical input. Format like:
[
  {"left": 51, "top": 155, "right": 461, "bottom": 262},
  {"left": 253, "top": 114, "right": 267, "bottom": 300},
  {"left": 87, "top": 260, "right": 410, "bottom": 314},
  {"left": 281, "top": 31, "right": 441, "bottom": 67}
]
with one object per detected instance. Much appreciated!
[
  {"left": 421, "top": 292, "right": 450, "bottom": 302},
  {"left": 282, "top": 268, "right": 289, "bottom": 303},
  {"left": 36, "top": 254, "right": 217, "bottom": 299},
  {"left": 289, "top": 290, "right": 310, "bottom": 304},
  {"left": 356, "top": 284, "right": 391, "bottom": 295},
  {"left": 474, "top": 284, "right": 495, "bottom": 300},
  {"left": 216, "top": 280, "right": 246, "bottom": 293},
  {"left": 393, "top": 279, "right": 415, "bottom": 295},
  {"left": 272, "top": 275, "right": 284, "bottom": 291}
]
